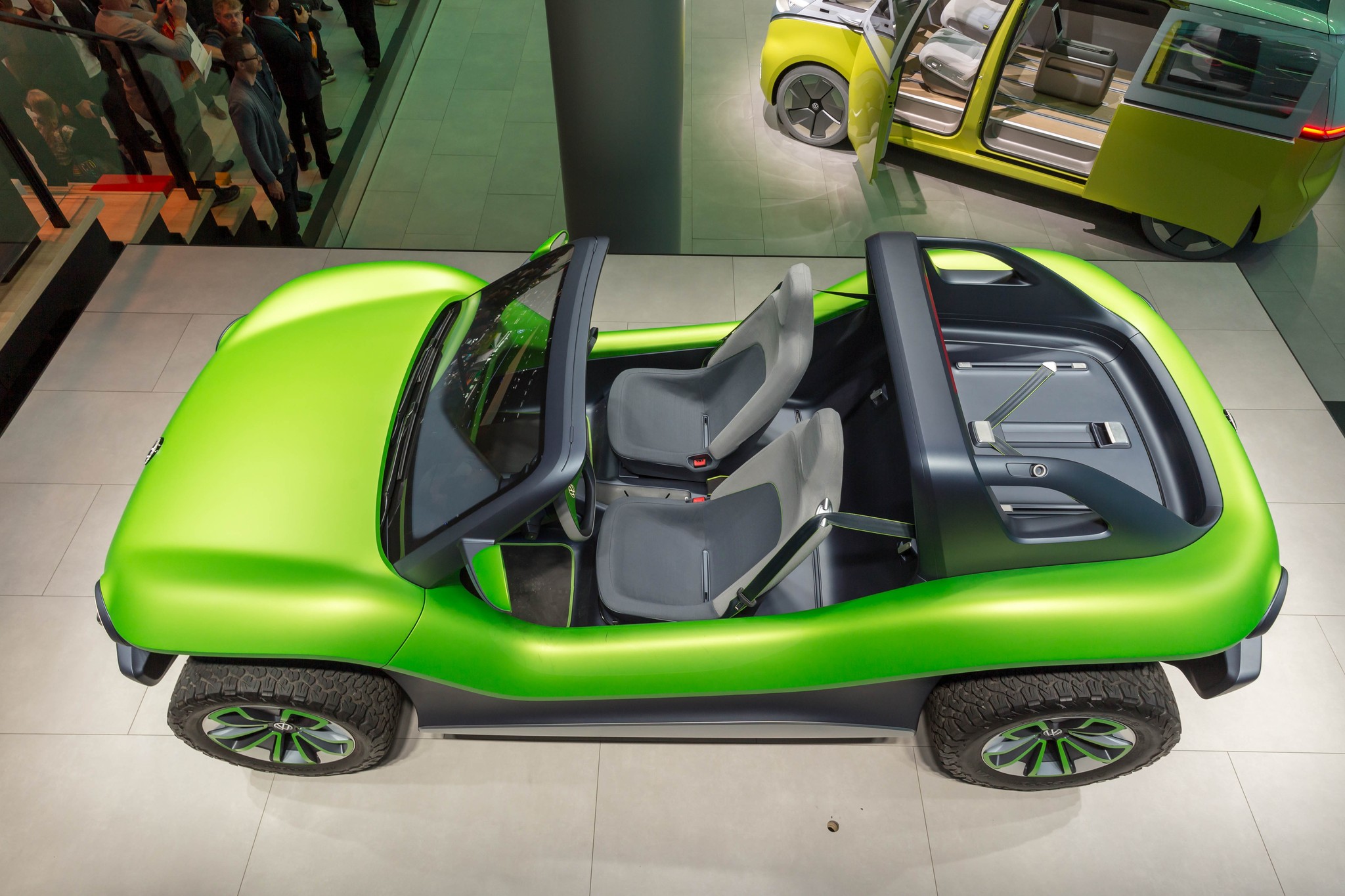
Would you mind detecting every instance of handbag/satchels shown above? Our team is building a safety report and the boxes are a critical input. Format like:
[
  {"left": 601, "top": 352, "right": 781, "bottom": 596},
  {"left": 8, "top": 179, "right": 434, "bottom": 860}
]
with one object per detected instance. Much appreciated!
[
  {"left": 167, "top": 10, "right": 212, "bottom": 84},
  {"left": 72, "top": 152, "right": 115, "bottom": 183},
  {"left": 160, "top": 22, "right": 199, "bottom": 89}
]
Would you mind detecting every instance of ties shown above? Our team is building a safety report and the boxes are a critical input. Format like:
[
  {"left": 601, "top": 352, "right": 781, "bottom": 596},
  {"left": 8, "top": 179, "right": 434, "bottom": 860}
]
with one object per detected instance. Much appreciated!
[{"left": 51, "top": 15, "right": 90, "bottom": 84}]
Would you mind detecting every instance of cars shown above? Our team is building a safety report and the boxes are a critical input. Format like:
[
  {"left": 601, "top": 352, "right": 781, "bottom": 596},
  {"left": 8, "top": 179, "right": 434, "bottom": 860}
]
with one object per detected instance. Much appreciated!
[
  {"left": 93, "top": 229, "right": 1296, "bottom": 793},
  {"left": 760, "top": 1, "right": 1345, "bottom": 265}
]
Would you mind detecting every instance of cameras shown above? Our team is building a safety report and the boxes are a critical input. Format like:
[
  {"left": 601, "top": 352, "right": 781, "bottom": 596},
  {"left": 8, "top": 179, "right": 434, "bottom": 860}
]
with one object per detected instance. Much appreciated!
[{"left": 289, "top": 3, "right": 312, "bottom": 19}]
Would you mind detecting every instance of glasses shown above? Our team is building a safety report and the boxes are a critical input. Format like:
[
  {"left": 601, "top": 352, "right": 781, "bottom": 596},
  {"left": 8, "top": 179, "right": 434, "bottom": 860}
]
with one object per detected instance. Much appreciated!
[{"left": 242, "top": 55, "right": 260, "bottom": 62}]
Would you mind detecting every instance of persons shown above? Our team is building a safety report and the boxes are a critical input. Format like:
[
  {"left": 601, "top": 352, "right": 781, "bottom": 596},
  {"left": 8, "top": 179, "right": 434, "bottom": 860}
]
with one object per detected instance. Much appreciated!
[
  {"left": 240, "top": 0, "right": 344, "bottom": 143},
  {"left": 96, "top": 0, "right": 241, "bottom": 207},
  {"left": 0, "top": 0, "right": 166, "bottom": 182},
  {"left": 338, "top": 0, "right": 383, "bottom": 79},
  {"left": 374, "top": 0, "right": 398, "bottom": 6},
  {"left": 220, "top": 35, "right": 304, "bottom": 247},
  {"left": 201, "top": 0, "right": 314, "bottom": 212},
  {"left": 247, "top": 0, "right": 335, "bottom": 178},
  {"left": 123, "top": 0, "right": 235, "bottom": 120}
]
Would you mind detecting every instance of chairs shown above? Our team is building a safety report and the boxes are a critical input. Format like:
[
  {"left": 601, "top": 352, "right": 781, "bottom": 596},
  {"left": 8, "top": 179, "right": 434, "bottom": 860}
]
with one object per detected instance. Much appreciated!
[
  {"left": 595, "top": 409, "right": 844, "bottom": 626},
  {"left": 607, "top": 264, "right": 814, "bottom": 481},
  {"left": 918, "top": 0, "right": 1043, "bottom": 99}
]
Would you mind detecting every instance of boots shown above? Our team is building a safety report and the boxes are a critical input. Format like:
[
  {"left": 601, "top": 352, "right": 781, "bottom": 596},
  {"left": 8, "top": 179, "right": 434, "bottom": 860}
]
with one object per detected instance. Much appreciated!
[{"left": 196, "top": 179, "right": 240, "bottom": 209}]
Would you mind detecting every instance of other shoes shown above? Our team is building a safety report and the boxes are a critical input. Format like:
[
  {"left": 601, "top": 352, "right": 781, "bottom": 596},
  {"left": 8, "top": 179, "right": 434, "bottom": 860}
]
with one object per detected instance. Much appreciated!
[
  {"left": 320, "top": 160, "right": 335, "bottom": 179},
  {"left": 326, "top": 127, "right": 343, "bottom": 141},
  {"left": 297, "top": 190, "right": 313, "bottom": 212},
  {"left": 215, "top": 160, "right": 234, "bottom": 172},
  {"left": 145, "top": 130, "right": 153, "bottom": 136},
  {"left": 300, "top": 151, "right": 312, "bottom": 171},
  {"left": 143, "top": 139, "right": 163, "bottom": 153},
  {"left": 321, "top": 59, "right": 333, "bottom": 70},
  {"left": 321, "top": 68, "right": 336, "bottom": 85},
  {"left": 208, "top": 101, "right": 228, "bottom": 119},
  {"left": 374, "top": 0, "right": 397, "bottom": 6},
  {"left": 368, "top": 67, "right": 378, "bottom": 77},
  {"left": 303, "top": 125, "right": 309, "bottom": 134},
  {"left": 322, "top": 5, "right": 333, "bottom": 11}
]
